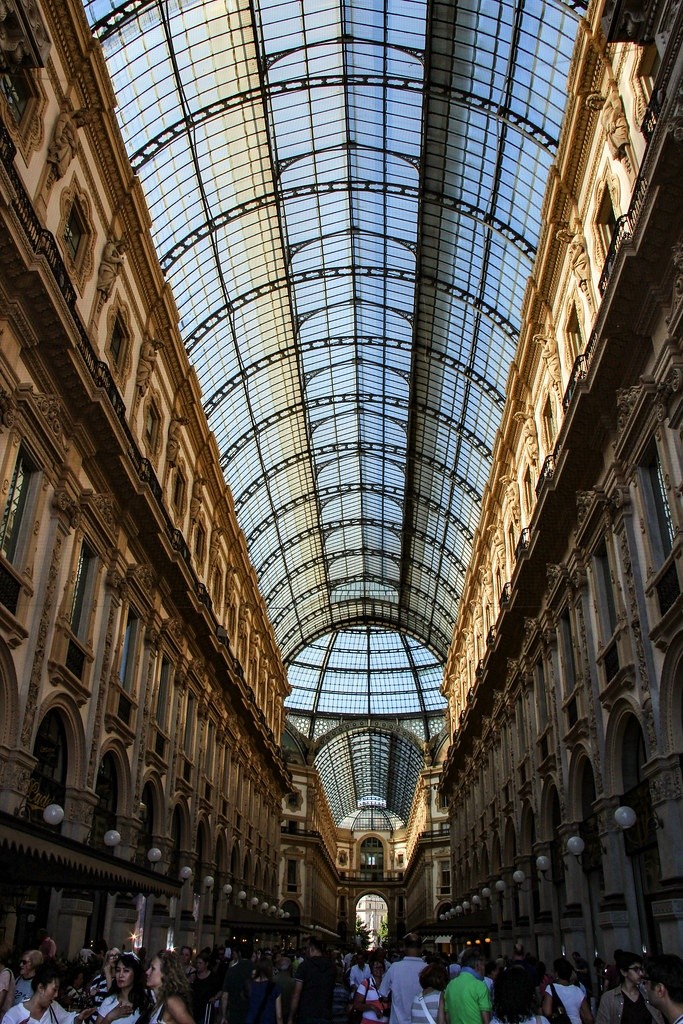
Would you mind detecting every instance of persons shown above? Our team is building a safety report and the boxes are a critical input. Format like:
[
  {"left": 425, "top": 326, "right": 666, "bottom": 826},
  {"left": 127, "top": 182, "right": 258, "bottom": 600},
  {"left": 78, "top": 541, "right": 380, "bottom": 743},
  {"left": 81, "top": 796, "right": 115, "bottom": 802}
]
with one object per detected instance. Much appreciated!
[{"left": 0, "top": 929, "right": 683, "bottom": 1024}]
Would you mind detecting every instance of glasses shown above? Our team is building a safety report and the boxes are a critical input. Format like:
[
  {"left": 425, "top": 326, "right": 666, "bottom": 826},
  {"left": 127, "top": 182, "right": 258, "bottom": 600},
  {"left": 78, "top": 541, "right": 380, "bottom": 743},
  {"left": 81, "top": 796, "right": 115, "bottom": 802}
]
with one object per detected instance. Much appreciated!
[
  {"left": 19, "top": 960, "right": 31, "bottom": 965},
  {"left": 627, "top": 966, "right": 643, "bottom": 972},
  {"left": 109, "top": 955, "right": 119, "bottom": 959},
  {"left": 640, "top": 976, "right": 668, "bottom": 992}
]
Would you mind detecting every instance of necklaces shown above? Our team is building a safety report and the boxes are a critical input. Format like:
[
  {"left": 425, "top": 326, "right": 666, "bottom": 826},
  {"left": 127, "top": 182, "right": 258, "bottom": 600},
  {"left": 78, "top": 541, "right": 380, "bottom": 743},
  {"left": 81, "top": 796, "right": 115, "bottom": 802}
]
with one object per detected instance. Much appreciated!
[{"left": 122, "top": 1000, "right": 129, "bottom": 1004}]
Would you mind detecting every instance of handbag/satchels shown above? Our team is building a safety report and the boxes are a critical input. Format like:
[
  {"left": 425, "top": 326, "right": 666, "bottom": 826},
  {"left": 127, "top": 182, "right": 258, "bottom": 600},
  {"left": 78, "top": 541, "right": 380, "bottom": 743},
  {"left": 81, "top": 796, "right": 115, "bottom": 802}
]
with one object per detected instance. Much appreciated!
[
  {"left": 547, "top": 983, "right": 572, "bottom": 1024},
  {"left": 349, "top": 978, "right": 370, "bottom": 1024},
  {"left": 379, "top": 998, "right": 392, "bottom": 1016}
]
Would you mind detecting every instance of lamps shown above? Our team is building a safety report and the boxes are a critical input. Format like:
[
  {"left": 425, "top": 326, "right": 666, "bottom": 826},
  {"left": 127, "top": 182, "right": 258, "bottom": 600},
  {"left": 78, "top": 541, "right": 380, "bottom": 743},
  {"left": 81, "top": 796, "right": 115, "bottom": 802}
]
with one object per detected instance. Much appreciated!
[
  {"left": 83, "top": 830, "right": 121, "bottom": 857},
  {"left": 615, "top": 799, "right": 664, "bottom": 849},
  {"left": 13, "top": 803, "right": 64, "bottom": 829},
  {"left": 566, "top": 835, "right": 607, "bottom": 866},
  {"left": 438, "top": 870, "right": 541, "bottom": 922},
  {"left": 535, "top": 856, "right": 568, "bottom": 882},
  {"left": 130, "top": 847, "right": 290, "bottom": 920}
]
